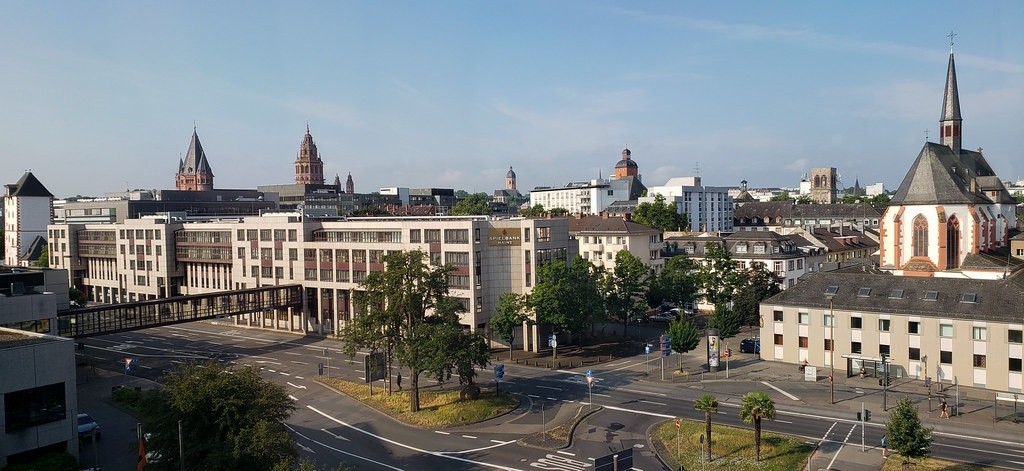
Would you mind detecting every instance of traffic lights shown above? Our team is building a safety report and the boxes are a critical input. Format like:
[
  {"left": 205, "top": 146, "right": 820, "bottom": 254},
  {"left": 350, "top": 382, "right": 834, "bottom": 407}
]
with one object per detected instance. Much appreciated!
[
  {"left": 879, "top": 379, "right": 882, "bottom": 386},
  {"left": 662, "top": 336, "right": 671, "bottom": 357},
  {"left": 864, "top": 410, "right": 871, "bottom": 422},
  {"left": 887, "top": 377, "right": 892, "bottom": 386},
  {"left": 857, "top": 413, "right": 861, "bottom": 421},
  {"left": 494, "top": 364, "right": 505, "bottom": 383}
]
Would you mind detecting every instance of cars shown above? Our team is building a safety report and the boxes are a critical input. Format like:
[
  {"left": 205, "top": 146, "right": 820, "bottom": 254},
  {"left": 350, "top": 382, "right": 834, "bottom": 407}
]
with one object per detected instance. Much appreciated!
[
  {"left": 740, "top": 339, "right": 760, "bottom": 353},
  {"left": 77, "top": 414, "right": 100, "bottom": 441}
]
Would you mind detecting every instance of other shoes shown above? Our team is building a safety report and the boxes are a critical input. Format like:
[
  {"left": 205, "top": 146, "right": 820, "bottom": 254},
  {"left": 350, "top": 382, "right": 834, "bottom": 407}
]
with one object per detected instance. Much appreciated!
[
  {"left": 945, "top": 417, "right": 950, "bottom": 419},
  {"left": 937, "top": 417, "right": 941, "bottom": 419},
  {"left": 398, "top": 387, "right": 402, "bottom": 391}
]
